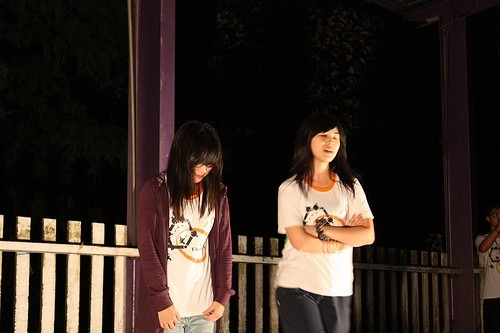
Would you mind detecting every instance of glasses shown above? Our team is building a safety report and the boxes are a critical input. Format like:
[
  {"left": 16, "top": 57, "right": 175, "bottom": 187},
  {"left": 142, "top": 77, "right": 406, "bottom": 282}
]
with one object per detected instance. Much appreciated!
[
  {"left": 195, "top": 163, "right": 213, "bottom": 168},
  {"left": 489, "top": 212, "right": 498, "bottom": 217}
]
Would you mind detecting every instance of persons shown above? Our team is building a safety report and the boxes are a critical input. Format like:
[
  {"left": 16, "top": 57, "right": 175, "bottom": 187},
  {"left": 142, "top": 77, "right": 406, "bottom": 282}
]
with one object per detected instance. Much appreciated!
[
  {"left": 274, "top": 112, "right": 376, "bottom": 333},
  {"left": 136, "top": 122, "right": 236, "bottom": 333},
  {"left": 475, "top": 204, "right": 500, "bottom": 333}
]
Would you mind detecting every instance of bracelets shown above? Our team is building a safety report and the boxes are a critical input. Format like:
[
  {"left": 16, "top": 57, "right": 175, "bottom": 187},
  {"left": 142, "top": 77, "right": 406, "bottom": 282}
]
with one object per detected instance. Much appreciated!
[{"left": 316, "top": 222, "right": 342, "bottom": 254}]
